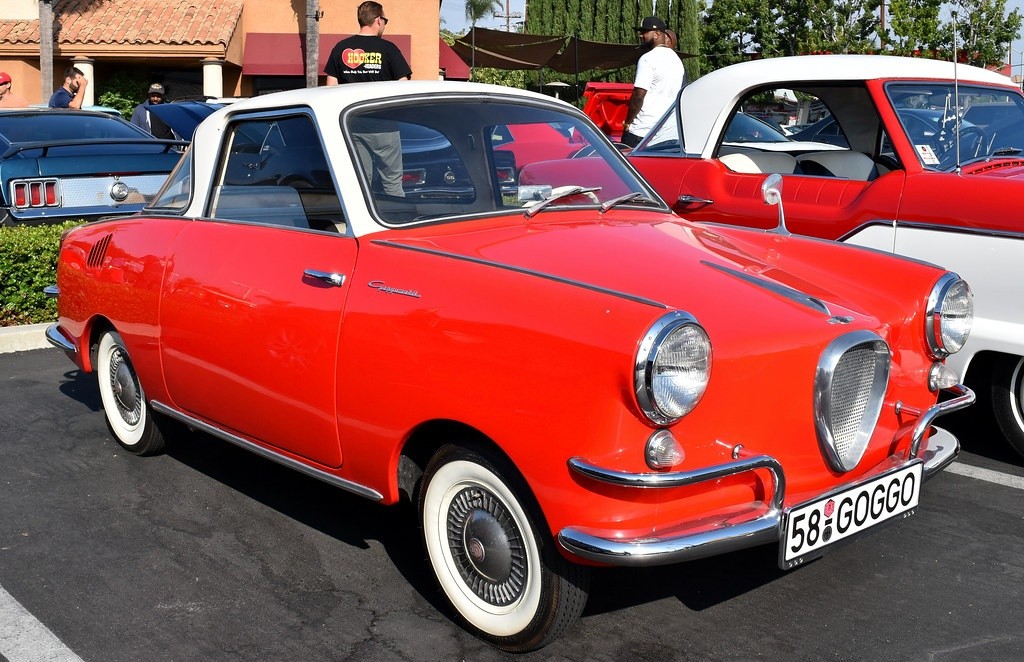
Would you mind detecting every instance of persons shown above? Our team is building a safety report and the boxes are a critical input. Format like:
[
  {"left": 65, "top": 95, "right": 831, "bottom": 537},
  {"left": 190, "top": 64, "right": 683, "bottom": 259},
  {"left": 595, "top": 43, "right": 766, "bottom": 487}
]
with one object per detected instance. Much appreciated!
[
  {"left": 130, "top": 83, "right": 182, "bottom": 152},
  {"left": 0, "top": 72, "right": 28, "bottom": 108},
  {"left": 619, "top": 16, "right": 686, "bottom": 150},
  {"left": 324, "top": 1, "right": 413, "bottom": 198},
  {"left": 49, "top": 66, "right": 88, "bottom": 109}
]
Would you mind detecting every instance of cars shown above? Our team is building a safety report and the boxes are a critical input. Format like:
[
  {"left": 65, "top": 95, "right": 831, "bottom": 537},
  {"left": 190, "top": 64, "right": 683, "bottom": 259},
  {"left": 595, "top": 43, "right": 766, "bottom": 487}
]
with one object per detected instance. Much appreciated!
[
  {"left": 45, "top": 81, "right": 976, "bottom": 653},
  {"left": 0, "top": 108, "right": 192, "bottom": 227},
  {"left": 717, "top": 100, "right": 1024, "bottom": 159},
  {"left": 143, "top": 97, "right": 596, "bottom": 196},
  {"left": 517, "top": 54, "right": 1023, "bottom": 468}
]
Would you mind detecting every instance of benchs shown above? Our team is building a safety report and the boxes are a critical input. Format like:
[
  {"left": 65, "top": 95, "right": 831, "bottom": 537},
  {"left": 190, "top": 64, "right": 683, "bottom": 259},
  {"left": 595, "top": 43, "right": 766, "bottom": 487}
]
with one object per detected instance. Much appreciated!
[{"left": 216, "top": 183, "right": 418, "bottom": 235}]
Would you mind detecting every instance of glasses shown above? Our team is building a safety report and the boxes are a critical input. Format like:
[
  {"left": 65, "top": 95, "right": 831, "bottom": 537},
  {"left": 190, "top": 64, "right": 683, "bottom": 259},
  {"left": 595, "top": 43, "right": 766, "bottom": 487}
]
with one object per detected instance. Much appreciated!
[{"left": 376, "top": 15, "right": 388, "bottom": 25}]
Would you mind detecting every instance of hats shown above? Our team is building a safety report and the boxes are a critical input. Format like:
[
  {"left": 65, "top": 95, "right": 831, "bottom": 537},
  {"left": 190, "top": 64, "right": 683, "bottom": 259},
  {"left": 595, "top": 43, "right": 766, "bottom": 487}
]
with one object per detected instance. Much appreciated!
[
  {"left": 0, "top": 72, "right": 11, "bottom": 85},
  {"left": 631, "top": 17, "right": 668, "bottom": 31},
  {"left": 148, "top": 84, "right": 165, "bottom": 95}
]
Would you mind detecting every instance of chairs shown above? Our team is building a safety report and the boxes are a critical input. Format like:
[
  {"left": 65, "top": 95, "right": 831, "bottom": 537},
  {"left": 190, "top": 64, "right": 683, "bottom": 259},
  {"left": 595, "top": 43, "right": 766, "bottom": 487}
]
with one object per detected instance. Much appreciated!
[{"left": 721, "top": 149, "right": 879, "bottom": 180}]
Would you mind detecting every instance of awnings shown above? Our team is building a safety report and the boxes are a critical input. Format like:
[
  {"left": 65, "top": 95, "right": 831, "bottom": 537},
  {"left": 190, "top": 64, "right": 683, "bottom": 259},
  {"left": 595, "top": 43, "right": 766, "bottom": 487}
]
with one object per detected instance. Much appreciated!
[
  {"left": 439, "top": 37, "right": 470, "bottom": 80},
  {"left": 243, "top": 33, "right": 411, "bottom": 76}
]
